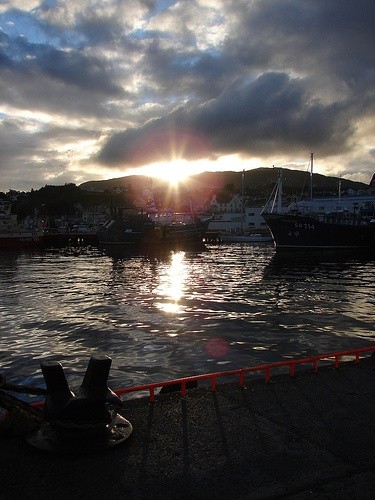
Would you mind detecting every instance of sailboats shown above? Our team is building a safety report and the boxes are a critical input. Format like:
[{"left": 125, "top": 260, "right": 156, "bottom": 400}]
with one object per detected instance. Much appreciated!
[{"left": 260, "top": 152, "right": 375, "bottom": 250}]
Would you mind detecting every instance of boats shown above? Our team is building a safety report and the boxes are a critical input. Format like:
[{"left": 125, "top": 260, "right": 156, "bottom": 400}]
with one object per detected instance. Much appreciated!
[
  {"left": 63, "top": 206, "right": 99, "bottom": 247},
  {"left": 205, "top": 229, "right": 273, "bottom": 243},
  {"left": 98, "top": 205, "right": 213, "bottom": 256},
  {"left": 0, "top": 189, "right": 65, "bottom": 249}
]
